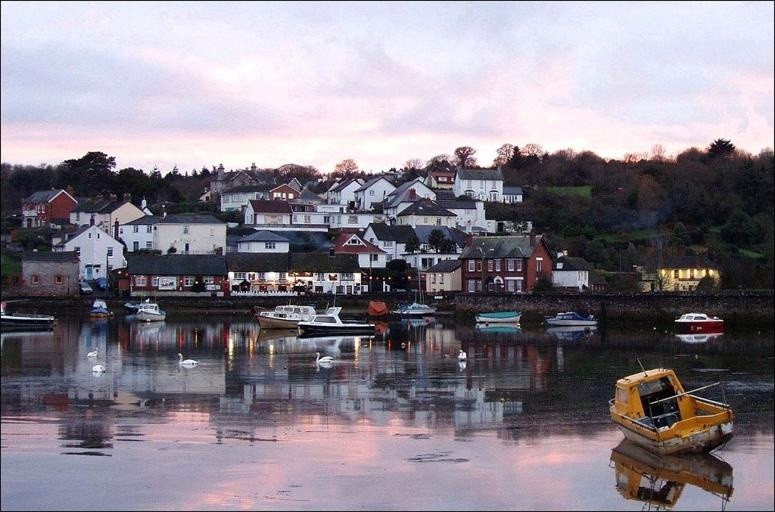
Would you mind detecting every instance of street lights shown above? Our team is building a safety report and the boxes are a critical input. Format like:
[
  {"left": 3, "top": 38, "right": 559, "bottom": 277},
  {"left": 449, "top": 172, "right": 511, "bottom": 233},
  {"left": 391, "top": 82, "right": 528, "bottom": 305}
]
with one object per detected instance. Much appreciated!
[{"left": 476, "top": 242, "right": 495, "bottom": 296}]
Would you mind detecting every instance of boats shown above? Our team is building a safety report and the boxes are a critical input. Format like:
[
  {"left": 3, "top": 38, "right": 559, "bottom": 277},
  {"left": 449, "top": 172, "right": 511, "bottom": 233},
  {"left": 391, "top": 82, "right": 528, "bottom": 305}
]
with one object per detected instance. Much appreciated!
[
  {"left": 675, "top": 312, "right": 724, "bottom": 328},
  {"left": 392, "top": 302, "right": 438, "bottom": 319},
  {"left": 254, "top": 304, "right": 376, "bottom": 333},
  {"left": 89, "top": 299, "right": 113, "bottom": 317},
  {"left": 608, "top": 358, "right": 735, "bottom": 455},
  {"left": 674, "top": 328, "right": 724, "bottom": 344},
  {"left": 124, "top": 301, "right": 166, "bottom": 322},
  {"left": 471, "top": 310, "right": 522, "bottom": 322},
  {"left": 544, "top": 312, "right": 598, "bottom": 325},
  {"left": 0, "top": 299, "right": 55, "bottom": 331},
  {"left": 544, "top": 326, "right": 599, "bottom": 340},
  {"left": 608, "top": 436, "right": 734, "bottom": 511},
  {"left": 474, "top": 323, "right": 521, "bottom": 334}
]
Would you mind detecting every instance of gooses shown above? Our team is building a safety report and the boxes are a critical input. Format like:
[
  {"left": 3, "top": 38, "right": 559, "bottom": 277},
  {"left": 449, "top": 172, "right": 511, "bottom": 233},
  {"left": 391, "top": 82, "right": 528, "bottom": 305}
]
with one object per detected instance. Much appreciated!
[
  {"left": 87, "top": 348, "right": 99, "bottom": 357},
  {"left": 315, "top": 352, "right": 334, "bottom": 363},
  {"left": 92, "top": 365, "right": 106, "bottom": 372},
  {"left": 457, "top": 349, "right": 467, "bottom": 362},
  {"left": 177, "top": 352, "right": 200, "bottom": 364}
]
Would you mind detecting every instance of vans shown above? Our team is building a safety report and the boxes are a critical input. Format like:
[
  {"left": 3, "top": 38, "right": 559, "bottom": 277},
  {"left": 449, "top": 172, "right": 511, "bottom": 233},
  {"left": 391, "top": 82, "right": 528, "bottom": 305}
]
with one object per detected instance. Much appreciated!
[{"left": 78, "top": 282, "right": 93, "bottom": 295}]
